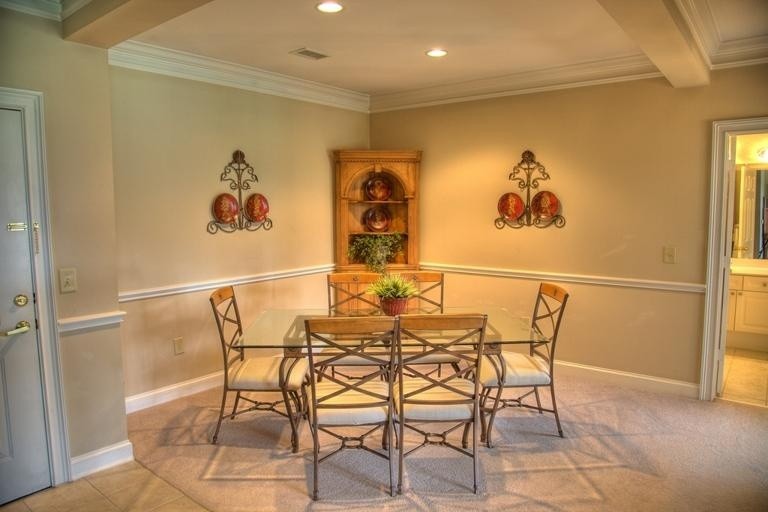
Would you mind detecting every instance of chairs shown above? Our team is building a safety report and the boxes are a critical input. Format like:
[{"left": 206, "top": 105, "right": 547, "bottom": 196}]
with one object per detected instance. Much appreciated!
[
  {"left": 470, "top": 281, "right": 569, "bottom": 448},
  {"left": 208, "top": 272, "right": 488, "bottom": 501}
]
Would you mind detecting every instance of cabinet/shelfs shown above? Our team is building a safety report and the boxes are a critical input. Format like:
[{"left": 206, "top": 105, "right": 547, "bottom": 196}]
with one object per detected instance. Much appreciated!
[
  {"left": 724, "top": 275, "right": 768, "bottom": 332},
  {"left": 326, "top": 146, "right": 426, "bottom": 341}
]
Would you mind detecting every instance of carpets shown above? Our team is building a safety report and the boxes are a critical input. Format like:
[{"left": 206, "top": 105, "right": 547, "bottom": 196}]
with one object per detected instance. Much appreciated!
[{"left": 127, "top": 367, "right": 766, "bottom": 512}]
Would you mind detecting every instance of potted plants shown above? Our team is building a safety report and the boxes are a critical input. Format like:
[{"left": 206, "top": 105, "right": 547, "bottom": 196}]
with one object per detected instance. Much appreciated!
[{"left": 342, "top": 231, "right": 406, "bottom": 274}]
